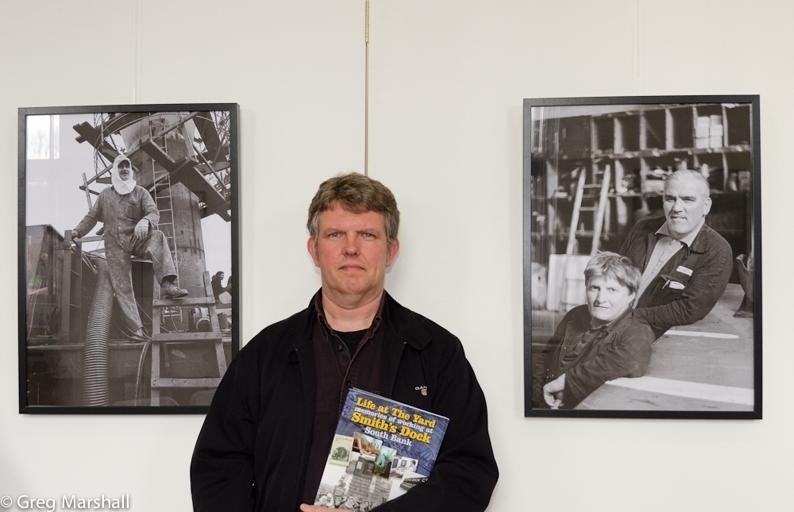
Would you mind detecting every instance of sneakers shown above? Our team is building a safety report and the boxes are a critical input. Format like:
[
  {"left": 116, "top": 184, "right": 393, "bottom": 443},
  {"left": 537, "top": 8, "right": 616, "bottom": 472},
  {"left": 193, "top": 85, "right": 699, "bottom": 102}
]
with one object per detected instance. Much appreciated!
[{"left": 160, "top": 281, "right": 188, "bottom": 300}]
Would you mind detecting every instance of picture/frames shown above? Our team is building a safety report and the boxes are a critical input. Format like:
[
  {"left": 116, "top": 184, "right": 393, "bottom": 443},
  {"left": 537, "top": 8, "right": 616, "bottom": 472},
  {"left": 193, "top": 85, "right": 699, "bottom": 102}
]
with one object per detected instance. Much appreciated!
[
  {"left": 523, "top": 94, "right": 763, "bottom": 420},
  {"left": 17, "top": 103, "right": 240, "bottom": 415}
]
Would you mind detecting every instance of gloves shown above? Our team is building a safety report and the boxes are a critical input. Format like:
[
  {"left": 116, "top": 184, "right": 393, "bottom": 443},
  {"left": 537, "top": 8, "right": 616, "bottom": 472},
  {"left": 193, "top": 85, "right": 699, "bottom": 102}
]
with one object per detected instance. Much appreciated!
[{"left": 134, "top": 218, "right": 149, "bottom": 240}]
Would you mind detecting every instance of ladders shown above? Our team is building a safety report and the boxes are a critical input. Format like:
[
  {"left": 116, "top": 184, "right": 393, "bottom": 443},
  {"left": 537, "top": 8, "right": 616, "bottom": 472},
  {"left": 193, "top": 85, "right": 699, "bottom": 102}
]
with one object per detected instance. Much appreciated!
[
  {"left": 149, "top": 118, "right": 227, "bottom": 407},
  {"left": 565, "top": 164, "right": 611, "bottom": 255},
  {"left": 58, "top": 230, "right": 82, "bottom": 343}
]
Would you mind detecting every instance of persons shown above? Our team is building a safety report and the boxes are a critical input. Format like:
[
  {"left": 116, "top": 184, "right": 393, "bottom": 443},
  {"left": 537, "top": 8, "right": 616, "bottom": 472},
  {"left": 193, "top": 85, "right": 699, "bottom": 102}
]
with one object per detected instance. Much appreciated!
[
  {"left": 189, "top": 170, "right": 502, "bottom": 512},
  {"left": 63, "top": 155, "right": 189, "bottom": 342},
  {"left": 618, "top": 166, "right": 735, "bottom": 342},
  {"left": 531, "top": 250, "right": 655, "bottom": 413}
]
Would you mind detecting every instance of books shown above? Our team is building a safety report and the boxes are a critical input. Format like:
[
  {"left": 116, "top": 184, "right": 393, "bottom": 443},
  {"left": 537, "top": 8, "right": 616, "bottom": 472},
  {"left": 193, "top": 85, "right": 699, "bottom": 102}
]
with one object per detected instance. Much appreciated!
[{"left": 312, "top": 385, "right": 450, "bottom": 509}]
